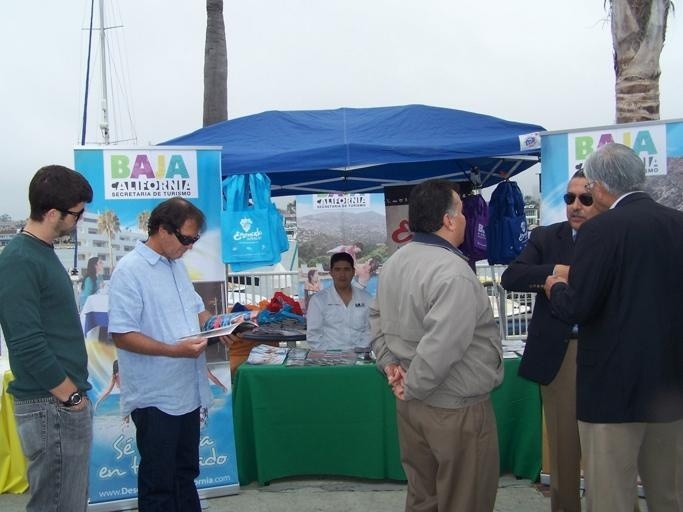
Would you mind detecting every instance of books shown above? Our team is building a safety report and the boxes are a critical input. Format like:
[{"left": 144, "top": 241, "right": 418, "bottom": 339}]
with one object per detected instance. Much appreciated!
[{"left": 176, "top": 307, "right": 264, "bottom": 345}]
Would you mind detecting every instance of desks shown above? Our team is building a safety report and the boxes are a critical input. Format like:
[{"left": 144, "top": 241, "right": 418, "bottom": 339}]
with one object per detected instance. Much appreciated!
[
  {"left": 242, "top": 322, "right": 306, "bottom": 347},
  {"left": 234, "top": 349, "right": 544, "bottom": 487}
]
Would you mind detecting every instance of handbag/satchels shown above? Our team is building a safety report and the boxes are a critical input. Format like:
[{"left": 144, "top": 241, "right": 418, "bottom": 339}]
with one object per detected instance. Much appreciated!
[
  {"left": 220, "top": 209, "right": 274, "bottom": 264},
  {"left": 231, "top": 203, "right": 289, "bottom": 272}
]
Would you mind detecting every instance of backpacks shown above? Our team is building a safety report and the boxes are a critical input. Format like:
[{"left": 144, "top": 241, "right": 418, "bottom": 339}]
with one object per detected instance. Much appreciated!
[
  {"left": 457, "top": 194, "right": 488, "bottom": 262},
  {"left": 483, "top": 178, "right": 528, "bottom": 265}
]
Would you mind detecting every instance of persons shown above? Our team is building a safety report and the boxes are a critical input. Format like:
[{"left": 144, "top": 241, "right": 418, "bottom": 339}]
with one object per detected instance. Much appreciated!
[
  {"left": 501, "top": 168, "right": 602, "bottom": 511},
  {"left": 94, "top": 359, "right": 130, "bottom": 425},
  {"left": 199, "top": 363, "right": 228, "bottom": 432},
  {"left": 542, "top": 142, "right": 683, "bottom": 512},
  {"left": 306, "top": 251, "right": 376, "bottom": 352},
  {"left": 366, "top": 178, "right": 508, "bottom": 512},
  {"left": 79, "top": 256, "right": 105, "bottom": 312},
  {"left": 329, "top": 243, "right": 363, "bottom": 261},
  {"left": 0, "top": 164, "right": 95, "bottom": 512},
  {"left": 107, "top": 195, "right": 242, "bottom": 512},
  {"left": 349, "top": 256, "right": 379, "bottom": 290},
  {"left": 304, "top": 268, "right": 321, "bottom": 299}
]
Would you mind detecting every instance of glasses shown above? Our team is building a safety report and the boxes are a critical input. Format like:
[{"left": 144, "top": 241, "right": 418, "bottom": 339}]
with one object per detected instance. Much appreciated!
[
  {"left": 563, "top": 194, "right": 592, "bottom": 206},
  {"left": 64, "top": 209, "right": 83, "bottom": 222},
  {"left": 584, "top": 182, "right": 596, "bottom": 193},
  {"left": 172, "top": 231, "right": 200, "bottom": 246}
]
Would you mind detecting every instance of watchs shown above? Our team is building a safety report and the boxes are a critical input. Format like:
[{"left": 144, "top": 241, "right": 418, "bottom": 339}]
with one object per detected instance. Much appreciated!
[{"left": 63, "top": 390, "right": 84, "bottom": 406}]
